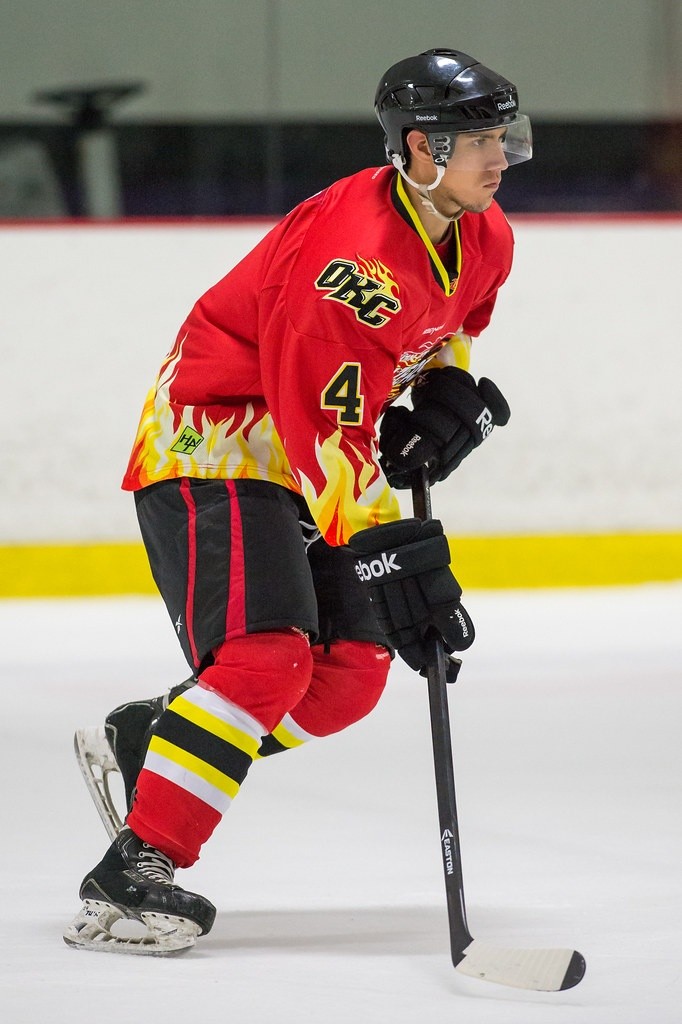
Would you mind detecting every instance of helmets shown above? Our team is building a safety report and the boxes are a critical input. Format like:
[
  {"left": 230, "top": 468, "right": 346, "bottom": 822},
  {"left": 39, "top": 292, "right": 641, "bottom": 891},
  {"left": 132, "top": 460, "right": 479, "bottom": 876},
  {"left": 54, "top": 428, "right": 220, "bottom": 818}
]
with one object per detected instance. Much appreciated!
[{"left": 374, "top": 47, "right": 519, "bottom": 159}]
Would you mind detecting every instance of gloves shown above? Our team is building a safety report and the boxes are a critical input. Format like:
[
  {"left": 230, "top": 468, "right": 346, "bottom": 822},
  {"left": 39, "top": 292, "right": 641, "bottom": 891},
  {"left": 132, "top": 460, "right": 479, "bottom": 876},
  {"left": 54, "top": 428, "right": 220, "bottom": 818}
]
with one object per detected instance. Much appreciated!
[
  {"left": 340, "top": 518, "right": 475, "bottom": 683},
  {"left": 378, "top": 365, "right": 511, "bottom": 490}
]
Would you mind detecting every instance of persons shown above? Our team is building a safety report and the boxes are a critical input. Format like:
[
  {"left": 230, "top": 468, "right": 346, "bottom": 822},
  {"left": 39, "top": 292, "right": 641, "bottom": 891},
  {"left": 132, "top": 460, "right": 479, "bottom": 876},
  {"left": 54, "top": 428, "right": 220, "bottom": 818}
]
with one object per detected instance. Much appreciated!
[{"left": 64, "top": 48, "right": 534, "bottom": 959}]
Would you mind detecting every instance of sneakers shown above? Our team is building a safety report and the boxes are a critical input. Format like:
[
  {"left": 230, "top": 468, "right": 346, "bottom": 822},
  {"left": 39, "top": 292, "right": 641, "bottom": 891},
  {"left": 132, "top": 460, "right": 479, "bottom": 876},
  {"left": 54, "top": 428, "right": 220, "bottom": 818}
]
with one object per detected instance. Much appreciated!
[
  {"left": 74, "top": 674, "right": 199, "bottom": 843},
  {"left": 63, "top": 787, "right": 216, "bottom": 958}
]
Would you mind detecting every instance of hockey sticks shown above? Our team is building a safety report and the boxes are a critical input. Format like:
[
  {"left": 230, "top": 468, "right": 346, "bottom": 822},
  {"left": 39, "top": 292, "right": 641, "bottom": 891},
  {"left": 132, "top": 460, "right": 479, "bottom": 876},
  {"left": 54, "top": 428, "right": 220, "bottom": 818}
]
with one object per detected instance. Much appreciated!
[{"left": 410, "top": 461, "right": 586, "bottom": 993}]
